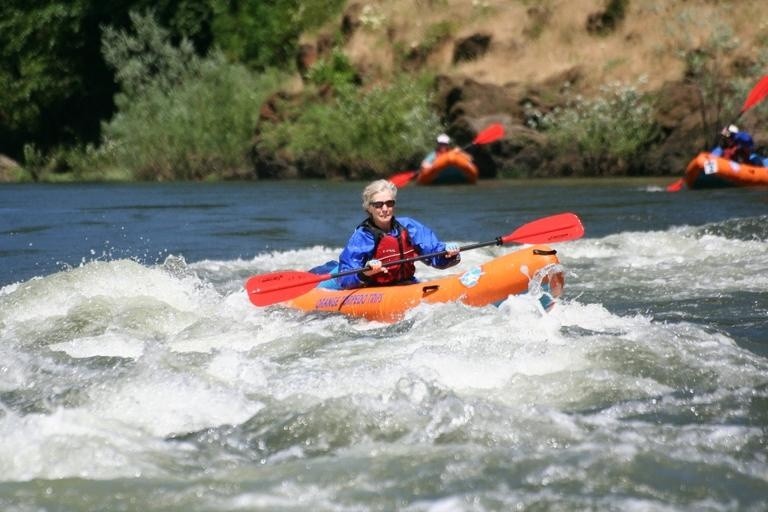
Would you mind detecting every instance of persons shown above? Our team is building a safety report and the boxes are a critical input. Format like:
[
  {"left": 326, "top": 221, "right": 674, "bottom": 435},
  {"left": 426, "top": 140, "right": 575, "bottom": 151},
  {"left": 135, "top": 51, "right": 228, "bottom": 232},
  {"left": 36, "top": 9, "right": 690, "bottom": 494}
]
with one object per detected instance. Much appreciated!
[
  {"left": 336, "top": 180, "right": 461, "bottom": 288},
  {"left": 712, "top": 124, "right": 763, "bottom": 166},
  {"left": 422, "top": 133, "right": 472, "bottom": 169}
]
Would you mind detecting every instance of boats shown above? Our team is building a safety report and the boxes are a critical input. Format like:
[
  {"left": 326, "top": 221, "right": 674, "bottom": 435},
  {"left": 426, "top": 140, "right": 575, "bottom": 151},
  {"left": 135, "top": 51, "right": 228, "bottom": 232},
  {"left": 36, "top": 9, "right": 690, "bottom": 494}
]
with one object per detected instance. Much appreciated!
[
  {"left": 416, "top": 152, "right": 478, "bottom": 185},
  {"left": 287, "top": 243, "right": 561, "bottom": 327},
  {"left": 684, "top": 152, "right": 768, "bottom": 188}
]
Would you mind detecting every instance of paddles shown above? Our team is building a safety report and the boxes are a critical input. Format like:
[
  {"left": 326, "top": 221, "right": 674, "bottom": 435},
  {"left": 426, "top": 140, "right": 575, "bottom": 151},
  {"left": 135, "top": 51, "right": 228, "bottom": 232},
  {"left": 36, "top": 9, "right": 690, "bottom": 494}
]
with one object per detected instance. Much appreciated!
[
  {"left": 390, "top": 124, "right": 505, "bottom": 187},
  {"left": 246, "top": 213, "right": 584, "bottom": 306},
  {"left": 666, "top": 77, "right": 767, "bottom": 191}
]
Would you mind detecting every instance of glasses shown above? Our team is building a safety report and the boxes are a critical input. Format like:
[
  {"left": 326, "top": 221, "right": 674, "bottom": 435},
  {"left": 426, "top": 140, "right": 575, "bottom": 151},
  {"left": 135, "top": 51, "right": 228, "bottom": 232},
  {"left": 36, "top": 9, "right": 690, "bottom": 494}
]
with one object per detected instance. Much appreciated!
[{"left": 370, "top": 200, "right": 395, "bottom": 208}]
